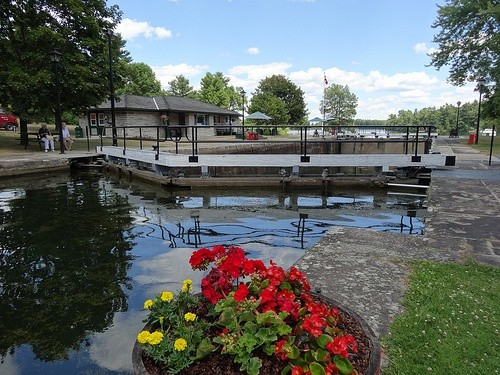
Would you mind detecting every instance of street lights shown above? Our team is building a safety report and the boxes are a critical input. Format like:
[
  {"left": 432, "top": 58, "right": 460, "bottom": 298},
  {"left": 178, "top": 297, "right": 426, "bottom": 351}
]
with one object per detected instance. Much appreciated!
[
  {"left": 456, "top": 101, "right": 462, "bottom": 137},
  {"left": 323, "top": 106, "right": 326, "bottom": 136},
  {"left": 239, "top": 89, "right": 247, "bottom": 140},
  {"left": 48, "top": 48, "right": 66, "bottom": 154},
  {"left": 474, "top": 77, "right": 486, "bottom": 145}
]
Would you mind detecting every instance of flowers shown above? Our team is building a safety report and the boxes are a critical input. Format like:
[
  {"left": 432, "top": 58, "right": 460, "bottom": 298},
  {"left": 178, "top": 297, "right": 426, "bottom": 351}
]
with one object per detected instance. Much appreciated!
[{"left": 137, "top": 244, "right": 362, "bottom": 375}]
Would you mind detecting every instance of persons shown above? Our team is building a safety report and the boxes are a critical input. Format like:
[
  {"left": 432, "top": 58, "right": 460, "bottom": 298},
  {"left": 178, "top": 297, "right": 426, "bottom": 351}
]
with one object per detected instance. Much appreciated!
[
  {"left": 312, "top": 128, "right": 356, "bottom": 137},
  {"left": 38, "top": 122, "right": 55, "bottom": 152},
  {"left": 62, "top": 122, "right": 73, "bottom": 151}
]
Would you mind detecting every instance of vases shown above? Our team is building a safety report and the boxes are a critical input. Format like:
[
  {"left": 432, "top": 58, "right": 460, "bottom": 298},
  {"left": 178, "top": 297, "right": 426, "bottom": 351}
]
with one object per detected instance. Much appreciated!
[{"left": 132, "top": 286, "right": 381, "bottom": 375}]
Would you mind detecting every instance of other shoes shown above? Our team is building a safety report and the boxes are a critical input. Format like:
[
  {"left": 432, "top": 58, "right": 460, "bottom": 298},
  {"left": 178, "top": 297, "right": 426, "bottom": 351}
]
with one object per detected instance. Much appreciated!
[
  {"left": 45, "top": 149, "right": 48, "bottom": 153},
  {"left": 51, "top": 149, "right": 55, "bottom": 152}
]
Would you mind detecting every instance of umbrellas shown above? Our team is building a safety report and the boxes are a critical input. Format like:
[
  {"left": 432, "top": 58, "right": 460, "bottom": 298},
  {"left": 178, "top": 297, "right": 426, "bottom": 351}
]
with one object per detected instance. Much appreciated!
[
  {"left": 247, "top": 112, "right": 272, "bottom": 140},
  {"left": 309, "top": 117, "right": 335, "bottom": 122}
]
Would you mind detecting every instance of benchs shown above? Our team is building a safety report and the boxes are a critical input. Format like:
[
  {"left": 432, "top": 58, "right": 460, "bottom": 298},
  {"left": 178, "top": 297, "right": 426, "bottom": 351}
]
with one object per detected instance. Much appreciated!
[
  {"left": 24, "top": 140, "right": 68, "bottom": 150},
  {"left": 14, "top": 137, "right": 59, "bottom": 146}
]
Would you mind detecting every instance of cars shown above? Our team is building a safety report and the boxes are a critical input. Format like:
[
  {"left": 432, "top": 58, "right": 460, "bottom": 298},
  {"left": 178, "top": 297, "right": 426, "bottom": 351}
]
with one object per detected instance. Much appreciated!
[{"left": 0, "top": 109, "right": 19, "bottom": 131}]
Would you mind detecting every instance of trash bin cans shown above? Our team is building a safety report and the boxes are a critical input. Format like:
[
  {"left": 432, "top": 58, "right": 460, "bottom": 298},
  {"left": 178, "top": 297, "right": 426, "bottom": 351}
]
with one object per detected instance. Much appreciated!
[
  {"left": 236, "top": 134, "right": 243, "bottom": 138},
  {"left": 74, "top": 126, "right": 83, "bottom": 138},
  {"left": 256, "top": 129, "right": 263, "bottom": 135},
  {"left": 246, "top": 131, "right": 257, "bottom": 140},
  {"left": 468, "top": 133, "right": 474, "bottom": 144},
  {"left": 272, "top": 129, "right": 276, "bottom": 135},
  {"left": 264, "top": 129, "right": 271, "bottom": 135}
]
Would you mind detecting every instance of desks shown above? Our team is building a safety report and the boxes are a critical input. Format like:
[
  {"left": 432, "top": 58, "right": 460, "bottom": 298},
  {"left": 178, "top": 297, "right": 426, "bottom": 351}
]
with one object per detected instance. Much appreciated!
[{"left": 18, "top": 131, "right": 60, "bottom": 138}]
